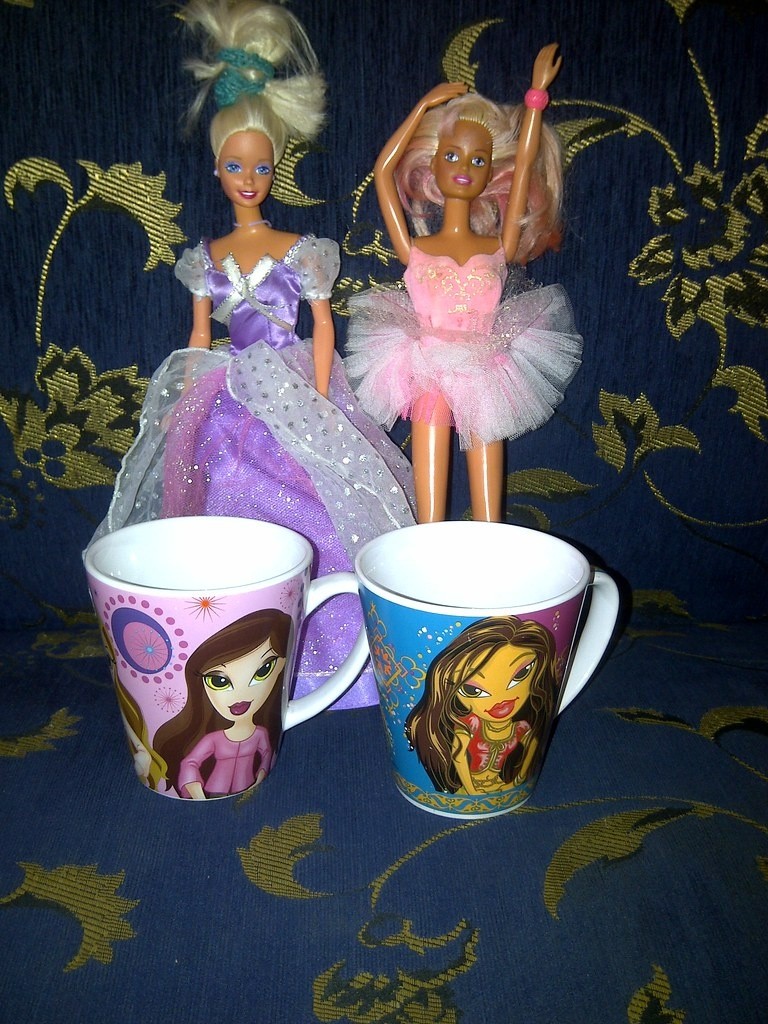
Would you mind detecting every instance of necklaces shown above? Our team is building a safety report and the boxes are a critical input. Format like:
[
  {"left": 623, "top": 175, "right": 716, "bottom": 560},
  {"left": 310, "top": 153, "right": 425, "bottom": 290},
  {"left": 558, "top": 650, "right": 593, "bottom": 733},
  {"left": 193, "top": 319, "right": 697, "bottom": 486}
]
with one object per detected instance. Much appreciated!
[{"left": 233, "top": 219, "right": 272, "bottom": 228}]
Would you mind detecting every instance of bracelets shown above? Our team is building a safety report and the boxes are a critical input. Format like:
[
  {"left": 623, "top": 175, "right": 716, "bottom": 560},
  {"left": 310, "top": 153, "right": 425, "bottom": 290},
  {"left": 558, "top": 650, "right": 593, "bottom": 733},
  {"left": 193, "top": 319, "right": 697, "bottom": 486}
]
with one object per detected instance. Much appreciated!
[{"left": 524, "top": 89, "right": 548, "bottom": 110}]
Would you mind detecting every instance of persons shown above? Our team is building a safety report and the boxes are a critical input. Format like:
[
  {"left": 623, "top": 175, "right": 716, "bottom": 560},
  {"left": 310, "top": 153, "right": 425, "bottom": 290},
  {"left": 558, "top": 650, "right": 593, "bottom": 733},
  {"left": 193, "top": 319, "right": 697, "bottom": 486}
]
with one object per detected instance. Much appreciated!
[
  {"left": 345, "top": 44, "right": 585, "bottom": 525},
  {"left": 85, "top": 1, "right": 419, "bottom": 709}
]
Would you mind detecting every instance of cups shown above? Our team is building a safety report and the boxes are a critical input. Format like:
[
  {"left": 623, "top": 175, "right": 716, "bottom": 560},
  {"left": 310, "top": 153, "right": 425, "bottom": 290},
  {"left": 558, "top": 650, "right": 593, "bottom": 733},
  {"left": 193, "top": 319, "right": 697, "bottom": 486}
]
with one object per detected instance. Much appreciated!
[
  {"left": 355, "top": 522, "right": 622, "bottom": 818},
  {"left": 85, "top": 518, "right": 368, "bottom": 803}
]
